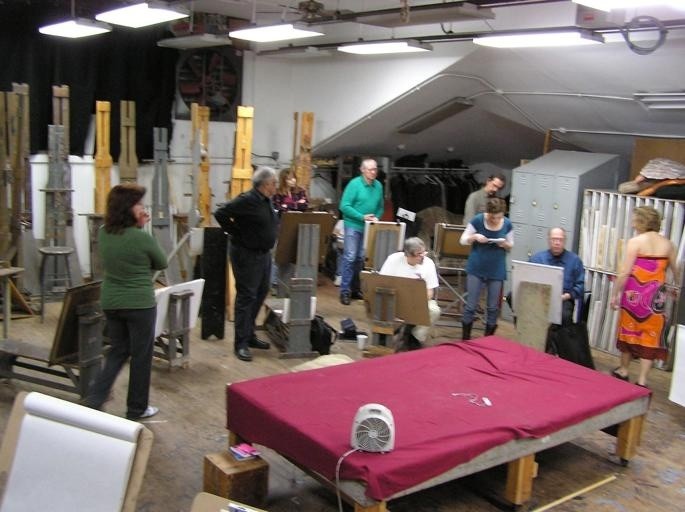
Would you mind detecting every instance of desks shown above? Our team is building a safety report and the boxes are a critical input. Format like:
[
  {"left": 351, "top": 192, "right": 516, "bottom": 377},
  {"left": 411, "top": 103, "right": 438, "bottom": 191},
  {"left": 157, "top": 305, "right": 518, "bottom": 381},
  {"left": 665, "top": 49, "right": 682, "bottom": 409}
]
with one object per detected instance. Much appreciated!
[{"left": 226, "top": 336, "right": 651, "bottom": 512}]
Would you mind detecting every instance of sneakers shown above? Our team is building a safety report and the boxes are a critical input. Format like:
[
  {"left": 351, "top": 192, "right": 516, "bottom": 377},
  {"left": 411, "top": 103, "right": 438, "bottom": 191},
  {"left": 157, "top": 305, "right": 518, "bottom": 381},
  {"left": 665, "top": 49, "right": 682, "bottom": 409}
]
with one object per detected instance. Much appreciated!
[
  {"left": 139, "top": 406, "right": 158, "bottom": 417},
  {"left": 334, "top": 276, "right": 342, "bottom": 286}
]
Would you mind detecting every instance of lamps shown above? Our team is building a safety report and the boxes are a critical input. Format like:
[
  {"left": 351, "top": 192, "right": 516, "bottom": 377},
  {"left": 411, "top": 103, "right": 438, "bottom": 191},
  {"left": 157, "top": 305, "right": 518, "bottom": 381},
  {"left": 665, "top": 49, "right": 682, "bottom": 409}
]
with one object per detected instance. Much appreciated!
[
  {"left": 38, "top": 0, "right": 112, "bottom": 39},
  {"left": 337, "top": 28, "right": 433, "bottom": 55},
  {"left": 229, "top": 1, "right": 325, "bottom": 43},
  {"left": 473, "top": 29, "right": 604, "bottom": 49},
  {"left": 95, "top": 0, "right": 191, "bottom": 29}
]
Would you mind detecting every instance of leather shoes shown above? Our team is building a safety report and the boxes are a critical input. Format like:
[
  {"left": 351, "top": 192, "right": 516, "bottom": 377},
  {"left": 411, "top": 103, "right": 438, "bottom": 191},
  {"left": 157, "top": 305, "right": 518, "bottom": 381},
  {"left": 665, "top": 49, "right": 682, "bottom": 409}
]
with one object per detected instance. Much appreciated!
[
  {"left": 234, "top": 348, "right": 252, "bottom": 361},
  {"left": 248, "top": 338, "right": 269, "bottom": 349},
  {"left": 341, "top": 294, "right": 350, "bottom": 305},
  {"left": 351, "top": 290, "right": 363, "bottom": 299}
]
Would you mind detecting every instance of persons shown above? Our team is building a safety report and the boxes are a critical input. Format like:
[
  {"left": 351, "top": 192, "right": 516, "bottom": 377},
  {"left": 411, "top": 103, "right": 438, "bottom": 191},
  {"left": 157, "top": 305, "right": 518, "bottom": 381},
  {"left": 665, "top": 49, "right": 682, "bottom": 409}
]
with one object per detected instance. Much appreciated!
[
  {"left": 507, "top": 227, "right": 594, "bottom": 368},
  {"left": 611, "top": 207, "right": 680, "bottom": 390},
  {"left": 84, "top": 182, "right": 168, "bottom": 420},
  {"left": 381, "top": 237, "right": 442, "bottom": 349},
  {"left": 338, "top": 158, "right": 385, "bottom": 305},
  {"left": 458, "top": 198, "right": 515, "bottom": 342},
  {"left": 464, "top": 174, "right": 505, "bottom": 225},
  {"left": 214, "top": 165, "right": 277, "bottom": 360},
  {"left": 270, "top": 168, "right": 308, "bottom": 296}
]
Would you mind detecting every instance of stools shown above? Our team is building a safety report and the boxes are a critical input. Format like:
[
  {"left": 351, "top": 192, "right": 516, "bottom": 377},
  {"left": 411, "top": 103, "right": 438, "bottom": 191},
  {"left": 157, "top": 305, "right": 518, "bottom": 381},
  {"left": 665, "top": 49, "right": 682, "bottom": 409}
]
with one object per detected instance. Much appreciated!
[{"left": 38, "top": 246, "right": 74, "bottom": 322}]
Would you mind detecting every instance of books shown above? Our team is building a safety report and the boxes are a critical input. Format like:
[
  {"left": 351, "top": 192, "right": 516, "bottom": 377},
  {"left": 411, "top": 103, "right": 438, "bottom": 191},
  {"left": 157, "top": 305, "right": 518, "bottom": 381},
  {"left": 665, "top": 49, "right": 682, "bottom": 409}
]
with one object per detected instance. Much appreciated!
[{"left": 227, "top": 444, "right": 255, "bottom": 461}]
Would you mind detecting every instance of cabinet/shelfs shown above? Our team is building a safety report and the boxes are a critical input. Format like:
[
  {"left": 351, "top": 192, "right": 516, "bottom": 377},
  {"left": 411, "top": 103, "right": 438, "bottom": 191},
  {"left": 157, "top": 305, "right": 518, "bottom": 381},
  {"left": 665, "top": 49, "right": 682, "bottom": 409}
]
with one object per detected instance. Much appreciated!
[
  {"left": 578, "top": 189, "right": 685, "bottom": 369},
  {"left": 501, "top": 149, "right": 620, "bottom": 319}
]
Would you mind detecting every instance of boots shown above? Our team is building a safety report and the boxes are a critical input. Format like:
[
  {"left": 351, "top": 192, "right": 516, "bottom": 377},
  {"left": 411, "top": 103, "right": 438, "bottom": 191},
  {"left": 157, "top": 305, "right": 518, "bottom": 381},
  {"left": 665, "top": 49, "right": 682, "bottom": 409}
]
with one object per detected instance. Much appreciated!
[
  {"left": 485, "top": 321, "right": 497, "bottom": 335},
  {"left": 462, "top": 321, "right": 472, "bottom": 339}
]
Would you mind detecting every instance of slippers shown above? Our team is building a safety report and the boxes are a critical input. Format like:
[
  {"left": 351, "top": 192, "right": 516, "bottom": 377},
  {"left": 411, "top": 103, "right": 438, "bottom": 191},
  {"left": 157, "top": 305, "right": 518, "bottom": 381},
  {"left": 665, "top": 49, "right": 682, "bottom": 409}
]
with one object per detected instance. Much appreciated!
[{"left": 612, "top": 367, "right": 628, "bottom": 380}]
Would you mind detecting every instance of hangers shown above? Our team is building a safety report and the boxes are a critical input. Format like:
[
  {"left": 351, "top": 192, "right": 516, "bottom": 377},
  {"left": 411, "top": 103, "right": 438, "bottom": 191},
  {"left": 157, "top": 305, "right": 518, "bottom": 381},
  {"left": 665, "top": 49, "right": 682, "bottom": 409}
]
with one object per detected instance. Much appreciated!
[{"left": 400, "top": 166, "right": 471, "bottom": 184}]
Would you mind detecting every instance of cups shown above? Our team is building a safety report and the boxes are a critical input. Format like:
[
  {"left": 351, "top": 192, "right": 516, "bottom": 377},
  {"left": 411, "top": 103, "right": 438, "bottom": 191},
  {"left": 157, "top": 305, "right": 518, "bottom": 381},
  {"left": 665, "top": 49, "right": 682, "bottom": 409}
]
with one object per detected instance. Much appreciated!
[{"left": 356, "top": 335, "right": 367, "bottom": 350}]
